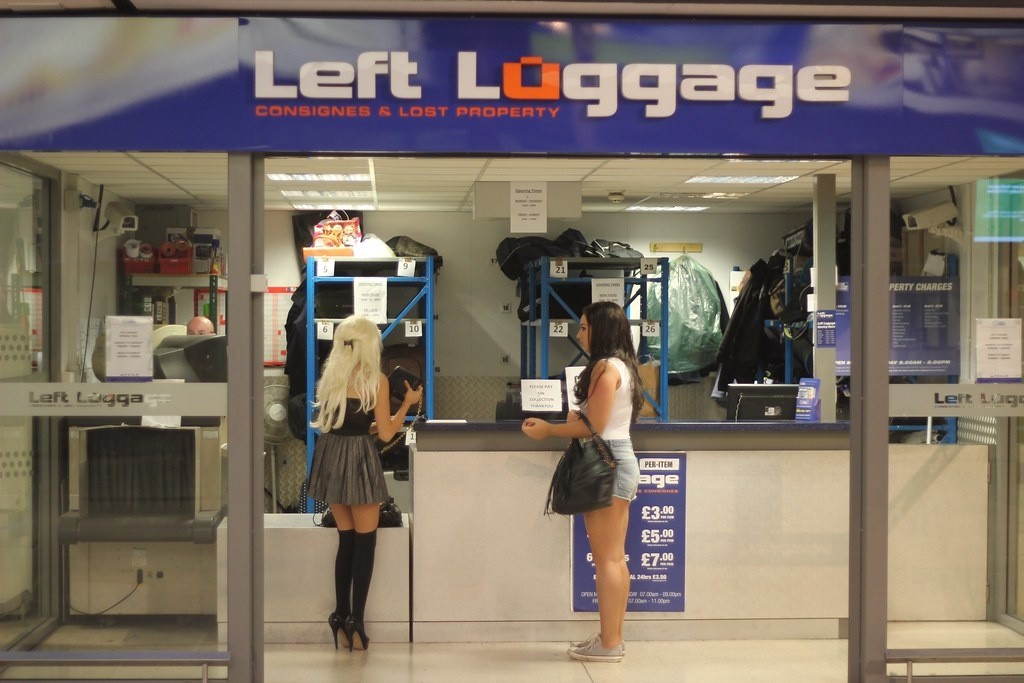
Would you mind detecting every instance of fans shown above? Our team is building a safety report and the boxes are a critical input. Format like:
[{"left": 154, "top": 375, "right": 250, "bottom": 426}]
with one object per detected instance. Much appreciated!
[{"left": 263, "top": 375, "right": 291, "bottom": 513}]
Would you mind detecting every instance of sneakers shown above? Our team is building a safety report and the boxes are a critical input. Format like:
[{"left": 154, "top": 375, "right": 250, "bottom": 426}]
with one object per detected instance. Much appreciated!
[{"left": 566, "top": 633, "right": 626, "bottom": 662}]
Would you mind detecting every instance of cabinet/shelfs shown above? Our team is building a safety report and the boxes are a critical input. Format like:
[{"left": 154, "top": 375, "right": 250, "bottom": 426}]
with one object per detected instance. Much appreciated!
[
  {"left": 521, "top": 254, "right": 669, "bottom": 420},
  {"left": 307, "top": 255, "right": 434, "bottom": 512}
]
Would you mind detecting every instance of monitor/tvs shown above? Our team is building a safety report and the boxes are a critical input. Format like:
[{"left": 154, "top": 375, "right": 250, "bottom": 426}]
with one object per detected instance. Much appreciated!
[
  {"left": 725, "top": 383, "right": 800, "bottom": 421},
  {"left": 151, "top": 334, "right": 229, "bottom": 384},
  {"left": 506, "top": 387, "right": 562, "bottom": 420}
]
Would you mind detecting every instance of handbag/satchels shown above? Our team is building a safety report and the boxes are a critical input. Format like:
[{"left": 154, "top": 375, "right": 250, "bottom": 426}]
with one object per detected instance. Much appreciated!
[
  {"left": 543, "top": 411, "right": 618, "bottom": 515},
  {"left": 496, "top": 227, "right": 601, "bottom": 282},
  {"left": 387, "top": 365, "right": 423, "bottom": 403}
]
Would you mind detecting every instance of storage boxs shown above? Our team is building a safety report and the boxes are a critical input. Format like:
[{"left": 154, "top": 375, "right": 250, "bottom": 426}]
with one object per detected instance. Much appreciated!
[{"left": 123, "top": 227, "right": 220, "bottom": 275}]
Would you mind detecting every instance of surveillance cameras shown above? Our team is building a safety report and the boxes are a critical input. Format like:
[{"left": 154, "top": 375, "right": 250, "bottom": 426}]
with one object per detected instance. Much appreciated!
[
  {"left": 903, "top": 201, "right": 958, "bottom": 230},
  {"left": 105, "top": 201, "right": 137, "bottom": 232}
]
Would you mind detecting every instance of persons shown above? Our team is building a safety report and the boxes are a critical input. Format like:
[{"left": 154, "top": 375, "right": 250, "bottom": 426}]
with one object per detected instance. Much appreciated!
[
  {"left": 307, "top": 315, "right": 423, "bottom": 652},
  {"left": 522, "top": 301, "right": 645, "bottom": 662},
  {"left": 187, "top": 316, "right": 214, "bottom": 336}
]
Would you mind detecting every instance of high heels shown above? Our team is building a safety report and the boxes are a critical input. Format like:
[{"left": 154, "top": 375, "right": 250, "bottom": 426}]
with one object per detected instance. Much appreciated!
[
  {"left": 344, "top": 616, "right": 369, "bottom": 652},
  {"left": 328, "top": 612, "right": 350, "bottom": 650}
]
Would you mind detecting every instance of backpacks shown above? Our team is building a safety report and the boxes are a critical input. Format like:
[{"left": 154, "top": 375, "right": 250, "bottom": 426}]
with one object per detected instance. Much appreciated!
[
  {"left": 385, "top": 235, "right": 440, "bottom": 275},
  {"left": 590, "top": 237, "right": 644, "bottom": 270}
]
